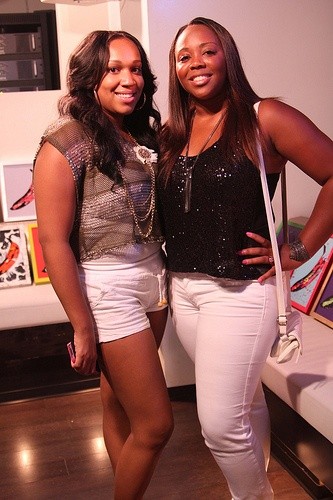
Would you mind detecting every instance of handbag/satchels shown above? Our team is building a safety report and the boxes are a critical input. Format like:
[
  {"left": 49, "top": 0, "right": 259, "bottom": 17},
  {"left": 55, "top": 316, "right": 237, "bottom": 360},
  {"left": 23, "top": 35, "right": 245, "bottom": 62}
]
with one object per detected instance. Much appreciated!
[{"left": 272, "top": 310, "right": 305, "bottom": 365}]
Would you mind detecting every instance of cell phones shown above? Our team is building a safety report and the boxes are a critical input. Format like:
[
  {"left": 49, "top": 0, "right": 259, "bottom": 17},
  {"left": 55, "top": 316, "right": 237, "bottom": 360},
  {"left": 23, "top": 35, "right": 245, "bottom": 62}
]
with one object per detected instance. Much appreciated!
[{"left": 67, "top": 342, "right": 101, "bottom": 374}]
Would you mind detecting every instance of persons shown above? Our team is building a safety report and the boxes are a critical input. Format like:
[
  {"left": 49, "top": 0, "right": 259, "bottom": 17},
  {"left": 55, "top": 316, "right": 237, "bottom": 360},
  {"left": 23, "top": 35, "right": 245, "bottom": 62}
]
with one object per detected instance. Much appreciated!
[
  {"left": 33, "top": 30, "right": 177, "bottom": 500},
  {"left": 163, "top": 17, "right": 333, "bottom": 500}
]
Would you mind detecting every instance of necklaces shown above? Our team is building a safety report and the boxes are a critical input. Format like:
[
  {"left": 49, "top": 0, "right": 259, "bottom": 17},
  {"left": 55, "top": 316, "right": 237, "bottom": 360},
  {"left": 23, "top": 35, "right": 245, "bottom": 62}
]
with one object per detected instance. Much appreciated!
[
  {"left": 181, "top": 106, "right": 227, "bottom": 212},
  {"left": 109, "top": 124, "right": 156, "bottom": 239}
]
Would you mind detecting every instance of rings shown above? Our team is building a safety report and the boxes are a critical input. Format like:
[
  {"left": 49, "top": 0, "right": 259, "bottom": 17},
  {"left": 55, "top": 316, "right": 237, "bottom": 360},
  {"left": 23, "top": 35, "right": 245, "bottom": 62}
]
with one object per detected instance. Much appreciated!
[{"left": 268, "top": 256, "right": 274, "bottom": 264}]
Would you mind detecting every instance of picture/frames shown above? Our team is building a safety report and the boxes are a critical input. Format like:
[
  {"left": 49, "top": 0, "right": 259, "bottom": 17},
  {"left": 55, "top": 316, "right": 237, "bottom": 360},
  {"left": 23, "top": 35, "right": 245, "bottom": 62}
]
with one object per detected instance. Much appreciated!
[
  {"left": 28, "top": 224, "right": 51, "bottom": 285},
  {"left": 310, "top": 263, "right": 333, "bottom": 328},
  {"left": 0, "top": 224, "right": 32, "bottom": 288},
  {"left": 276, "top": 221, "right": 333, "bottom": 315},
  {"left": 0, "top": 161, "right": 37, "bottom": 222}
]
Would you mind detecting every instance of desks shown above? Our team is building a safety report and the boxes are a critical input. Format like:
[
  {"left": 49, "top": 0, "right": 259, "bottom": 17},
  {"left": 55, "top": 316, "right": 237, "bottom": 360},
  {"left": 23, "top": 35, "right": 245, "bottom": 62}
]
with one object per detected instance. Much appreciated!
[{"left": 261, "top": 308, "right": 332, "bottom": 500}]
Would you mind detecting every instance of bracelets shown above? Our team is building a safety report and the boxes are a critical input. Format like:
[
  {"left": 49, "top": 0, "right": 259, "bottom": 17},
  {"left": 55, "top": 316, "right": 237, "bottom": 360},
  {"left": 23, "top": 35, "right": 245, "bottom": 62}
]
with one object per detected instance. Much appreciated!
[{"left": 291, "top": 238, "right": 310, "bottom": 261}]
